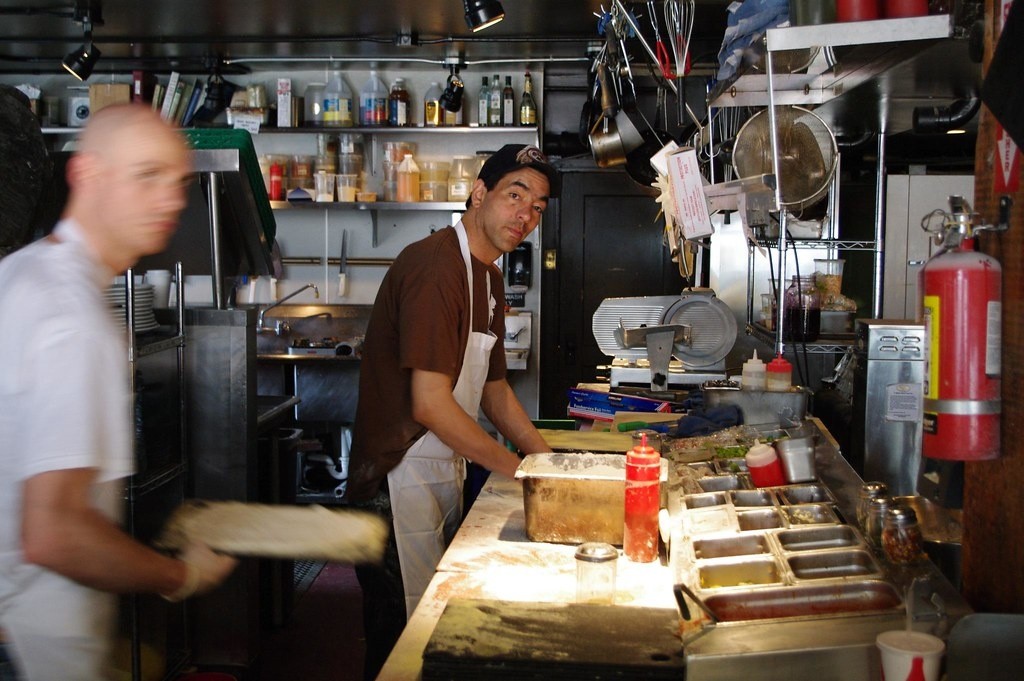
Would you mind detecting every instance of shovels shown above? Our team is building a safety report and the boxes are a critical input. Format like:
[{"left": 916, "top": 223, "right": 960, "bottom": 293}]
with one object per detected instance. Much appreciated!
[{"left": 618, "top": 420, "right": 679, "bottom": 438}]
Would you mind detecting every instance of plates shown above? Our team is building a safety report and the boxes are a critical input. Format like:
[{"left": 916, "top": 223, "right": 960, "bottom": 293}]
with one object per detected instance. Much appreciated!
[{"left": 103, "top": 283, "right": 161, "bottom": 336}]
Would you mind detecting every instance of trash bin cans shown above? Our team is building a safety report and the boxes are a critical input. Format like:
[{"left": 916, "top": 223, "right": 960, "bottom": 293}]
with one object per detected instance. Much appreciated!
[{"left": 255, "top": 395, "right": 304, "bottom": 629}]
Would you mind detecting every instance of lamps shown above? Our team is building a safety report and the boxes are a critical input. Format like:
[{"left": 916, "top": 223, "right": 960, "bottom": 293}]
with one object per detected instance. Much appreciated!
[
  {"left": 62, "top": 0, "right": 105, "bottom": 82},
  {"left": 463, "top": 0, "right": 505, "bottom": 33}
]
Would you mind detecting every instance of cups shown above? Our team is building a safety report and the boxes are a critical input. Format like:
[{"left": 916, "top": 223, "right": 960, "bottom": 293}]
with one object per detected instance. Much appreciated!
[
  {"left": 145, "top": 270, "right": 172, "bottom": 309},
  {"left": 313, "top": 173, "right": 335, "bottom": 203},
  {"left": 759, "top": 277, "right": 792, "bottom": 329},
  {"left": 815, "top": 258, "right": 845, "bottom": 307},
  {"left": 875, "top": 629, "right": 946, "bottom": 681},
  {"left": 336, "top": 174, "right": 358, "bottom": 203}
]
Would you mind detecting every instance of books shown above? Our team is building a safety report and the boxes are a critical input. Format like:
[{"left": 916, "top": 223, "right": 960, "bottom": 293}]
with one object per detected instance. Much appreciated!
[{"left": 133, "top": 71, "right": 209, "bottom": 128}]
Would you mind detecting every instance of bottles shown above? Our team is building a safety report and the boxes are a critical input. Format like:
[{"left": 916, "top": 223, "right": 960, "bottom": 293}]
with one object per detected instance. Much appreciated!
[
  {"left": 866, "top": 496, "right": 896, "bottom": 555},
  {"left": 784, "top": 275, "right": 822, "bottom": 343},
  {"left": 767, "top": 351, "right": 792, "bottom": 392},
  {"left": 475, "top": 150, "right": 495, "bottom": 178},
  {"left": 880, "top": 505, "right": 922, "bottom": 572},
  {"left": 396, "top": 153, "right": 420, "bottom": 202},
  {"left": 303, "top": 70, "right": 538, "bottom": 129},
  {"left": 745, "top": 438, "right": 786, "bottom": 488},
  {"left": 270, "top": 161, "right": 283, "bottom": 201},
  {"left": 856, "top": 481, "right": 886, "bottom": 534},
  {"left": 742, "top": 349, "right": 767, "bottom": 392},
  {"left": 447, "top": 155, "right": 473, "bottom": 202},
  {"left": 622, "top": 433, "right": 660, "bottom": 564}
]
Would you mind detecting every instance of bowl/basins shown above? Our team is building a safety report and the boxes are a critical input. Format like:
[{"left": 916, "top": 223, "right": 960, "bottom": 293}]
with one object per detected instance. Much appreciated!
[{"left": 289, "top": 131, "right": 448, "bottom": 203}]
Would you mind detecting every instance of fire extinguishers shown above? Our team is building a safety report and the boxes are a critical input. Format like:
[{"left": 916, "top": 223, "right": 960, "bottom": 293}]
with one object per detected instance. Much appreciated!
[{"left": 917, "top": 193, "right": 1013, "bottom": 463}]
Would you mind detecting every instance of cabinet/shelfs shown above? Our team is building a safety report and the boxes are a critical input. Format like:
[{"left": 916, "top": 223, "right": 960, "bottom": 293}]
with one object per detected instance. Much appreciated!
[{"left": 39, "top": 57, "right": 547, "bottom": 678}]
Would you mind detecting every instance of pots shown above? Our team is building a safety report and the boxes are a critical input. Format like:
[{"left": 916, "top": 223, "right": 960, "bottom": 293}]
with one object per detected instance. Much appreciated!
[{"left": 587, "top": 29, "right": 680, "bottom": 197}]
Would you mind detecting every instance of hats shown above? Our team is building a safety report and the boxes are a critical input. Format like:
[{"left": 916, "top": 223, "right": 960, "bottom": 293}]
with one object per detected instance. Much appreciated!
[{"left": 486, "top": 143, "right": 561, "bottom": 199}]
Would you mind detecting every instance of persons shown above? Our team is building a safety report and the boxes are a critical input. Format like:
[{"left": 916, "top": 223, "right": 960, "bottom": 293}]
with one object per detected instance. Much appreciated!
[
  {"left": 0, "top": 105, "right": 235, "bottom": 681},
  {"left": 346, "top": 144, "right": 563, "bottom": 681}
]
